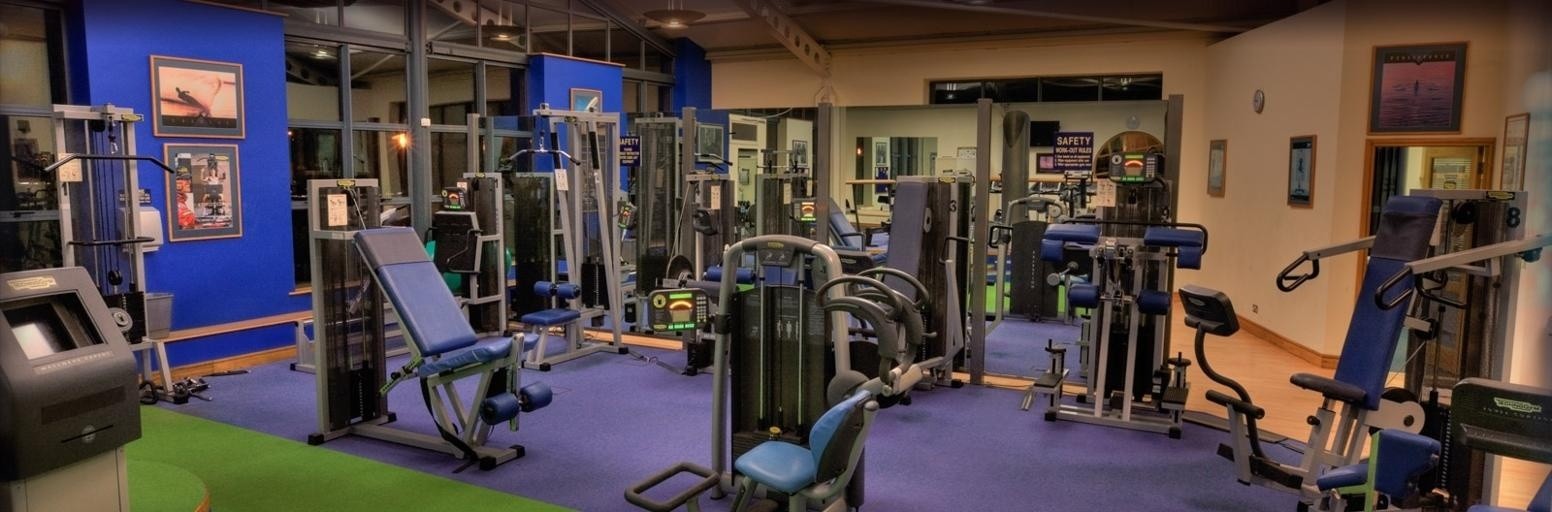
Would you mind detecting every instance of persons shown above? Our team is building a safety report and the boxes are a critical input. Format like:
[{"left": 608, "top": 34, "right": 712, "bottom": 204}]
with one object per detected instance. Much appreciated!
[{"left": 175, "top": 153, "right": 233, "bottom": 230}]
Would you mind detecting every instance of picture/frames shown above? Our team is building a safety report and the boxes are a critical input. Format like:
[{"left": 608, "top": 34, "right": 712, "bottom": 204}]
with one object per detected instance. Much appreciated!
[
  {"left": 1285, "top": 133, "right": 1317, "bottom": 209},
  {"left": 1206, "top": 138, "right": 1228, "bottom": 197},
  {"left": 1498, "top": 113, "right": 1531, "bottom": 191},
  {"left": 1368, "top": 40, "right": 1469, "bottom": 135},
  {"left": 791, "top": 139, "right": 808, "bottom": 166},
  {"left": 146, "top": 52, "right": 248, "bottom": 141},
  {"left": 570, "top": 87, "right": 604, "bottom": 114},
  {"left": 956, "top": 147, "right": 977, "bottom": 158},
  {"left": 696, "top": 123, "right": 725, "bottom": 164},
  {"left": 163, "top": 142, "right": 243, "bottom": 241},
  {"left": 1035, "top": 152, "right": 1065, "bottom": 174},
  {"left": 1, "top": 103, "right": 60, "bottom": 221},
  {"left": 874, "top": 142, "right": 889, "bottom": 194}
]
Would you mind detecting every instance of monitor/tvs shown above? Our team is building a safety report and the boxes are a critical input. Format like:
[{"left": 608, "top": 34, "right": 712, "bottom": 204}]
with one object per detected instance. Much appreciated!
[{"left": 1031, "top": 119, "right": 1061, "bottom": 147}]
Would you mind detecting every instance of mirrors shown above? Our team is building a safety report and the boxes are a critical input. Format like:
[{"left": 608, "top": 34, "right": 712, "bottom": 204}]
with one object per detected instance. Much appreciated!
[{"left": 855, "top": 137, "right": 937, "bottom": 212}]
